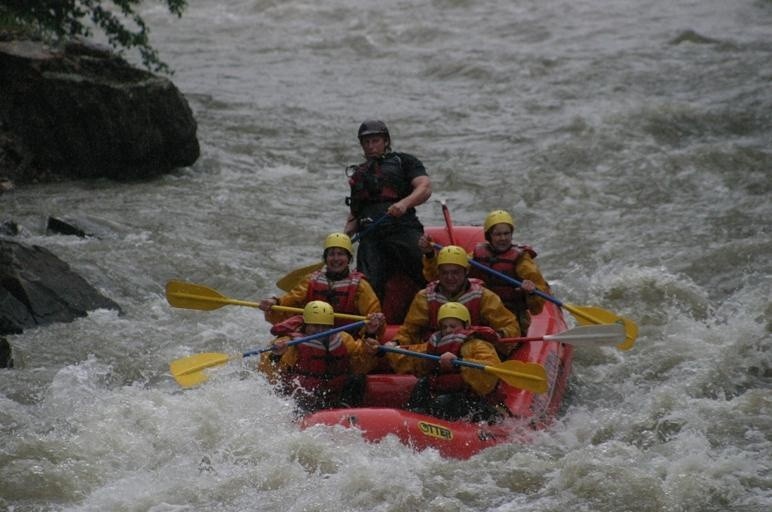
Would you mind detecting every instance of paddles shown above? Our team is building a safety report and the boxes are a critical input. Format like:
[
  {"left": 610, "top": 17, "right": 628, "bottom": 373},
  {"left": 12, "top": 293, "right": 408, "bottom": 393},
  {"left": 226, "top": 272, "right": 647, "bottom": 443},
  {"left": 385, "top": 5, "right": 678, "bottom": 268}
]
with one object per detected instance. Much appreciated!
[
  {"left": 374, "top": 345, "right": 548, "bottom": 394},
  {"left": 393, "top": 324, "right": 626, "bottom": 351},
  {"left": 168, "top": 322, "right": 365, "bottom": 389},
  {"left": 422, "top": 237, "right": 638, "bottom": 350},
  {"left": 165, "top": 278, "right": 365, "bottom": 320},
  {"left": 276, "top": 209, "right": 391, "bottom": 293}
]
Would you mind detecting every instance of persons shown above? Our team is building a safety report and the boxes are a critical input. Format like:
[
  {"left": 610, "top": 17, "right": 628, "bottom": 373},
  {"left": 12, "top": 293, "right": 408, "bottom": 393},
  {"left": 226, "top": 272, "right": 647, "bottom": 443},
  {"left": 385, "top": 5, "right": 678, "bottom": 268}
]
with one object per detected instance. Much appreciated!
[
  {"left": 344, "top": 117, "right": 432, "bottom": 307},
  {"left": 259, "top": 232, "right": 387, "bottom": 328},
  {"left": 361, "top": 301, "right": 501, "bottom": 425},
  {"left": 418, "top": 208, "right": 549, "bottom": 348},
  {"left": 259, "top": 300, "right": 379, "bottom": 415},
  {"left": 383, "top": 245, "right": 520, "bottom": 360}
]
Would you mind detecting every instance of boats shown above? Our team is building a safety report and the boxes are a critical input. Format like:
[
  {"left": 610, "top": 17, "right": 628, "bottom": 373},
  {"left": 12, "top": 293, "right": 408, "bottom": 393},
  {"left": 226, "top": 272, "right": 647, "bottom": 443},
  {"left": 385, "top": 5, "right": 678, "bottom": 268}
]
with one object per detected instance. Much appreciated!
[{"left": 275, "top": 223, "right": 574, "bottom": 462}]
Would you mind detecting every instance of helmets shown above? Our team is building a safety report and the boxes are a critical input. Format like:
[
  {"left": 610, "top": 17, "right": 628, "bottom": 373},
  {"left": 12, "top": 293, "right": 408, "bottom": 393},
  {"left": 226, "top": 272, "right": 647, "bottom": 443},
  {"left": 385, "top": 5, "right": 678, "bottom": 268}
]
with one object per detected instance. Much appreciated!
[
  {"left": 324, "top": 232, "right": 353, "bottom": 254},
  {"left": 484, "top": 210, "right": 514, "bottom": 231},
  {"left": 437, "top": 302, "right": 472, "bottom": 325},
  {"left": 437, "top": 245, "right": 470, "bottom": 270},
  {"left": 304, "top": 300, "right": 334, "bottom": 326},
  {"left": 358, "top": 120, "right": 387, "bottom": 136}
]
